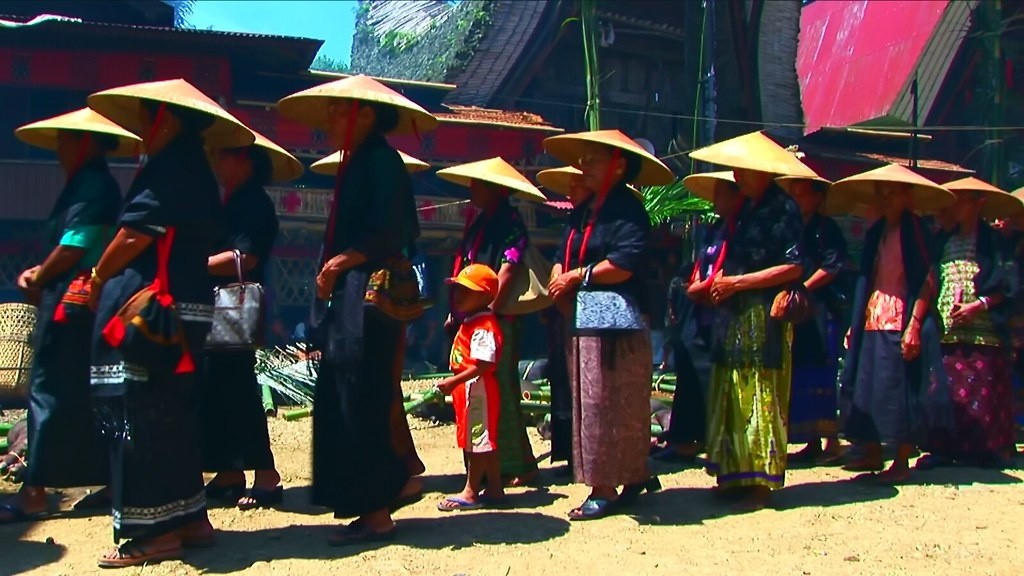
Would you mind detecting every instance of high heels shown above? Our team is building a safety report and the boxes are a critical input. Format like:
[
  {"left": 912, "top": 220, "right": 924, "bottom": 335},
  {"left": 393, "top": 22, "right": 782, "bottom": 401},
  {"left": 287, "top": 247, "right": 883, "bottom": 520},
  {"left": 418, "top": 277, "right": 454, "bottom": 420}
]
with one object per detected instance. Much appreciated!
[
  {"left": 621, "top": 477, "right": 662, "bottom": 501},
  {"left": 569, "top": 495, "right": 625, "bottom": 521}
]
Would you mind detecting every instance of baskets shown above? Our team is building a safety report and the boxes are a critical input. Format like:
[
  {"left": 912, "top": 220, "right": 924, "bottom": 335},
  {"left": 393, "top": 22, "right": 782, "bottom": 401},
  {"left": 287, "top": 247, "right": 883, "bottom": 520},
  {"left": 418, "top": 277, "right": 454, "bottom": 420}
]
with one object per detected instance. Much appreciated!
[{"left": 0, "top": 304, "right": 39, "bottom": 409}]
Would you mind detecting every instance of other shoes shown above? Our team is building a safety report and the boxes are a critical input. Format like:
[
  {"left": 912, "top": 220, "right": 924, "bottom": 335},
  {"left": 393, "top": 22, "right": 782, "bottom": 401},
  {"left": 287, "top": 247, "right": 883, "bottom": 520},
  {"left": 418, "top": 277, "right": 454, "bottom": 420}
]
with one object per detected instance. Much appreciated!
[
  {"left": 651, "top": 434, "right": 766, "bottom": 510},
  {"left": 787, "top": 445, "right": 1017, "bottom": 487}
]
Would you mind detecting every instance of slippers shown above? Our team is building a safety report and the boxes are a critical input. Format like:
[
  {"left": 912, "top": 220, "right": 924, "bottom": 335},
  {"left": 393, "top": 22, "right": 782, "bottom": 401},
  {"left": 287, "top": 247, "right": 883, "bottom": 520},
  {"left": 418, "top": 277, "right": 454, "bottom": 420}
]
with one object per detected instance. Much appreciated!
[
  {"left": 330, "top": 521, "right": 397, "bottom": 548},
  {"left": 97, "top": 540, "right": 183, "bottom": 568},
  {"left": 438, "top": 497, "right": 487, "bottom": 510},
  {"left": 479, "top": 494, "right": 507, "bottom": 504},
  {"left": 206, "top": 481, "right": 239, "bottom": 507},
  {"left": 239, "top": 486, "right": 284, "bottom": 511},
  {"left": 74, "top": 494, "right": 113, "bottom": 514},
  {"left": 0, "top": 495, "right": 51, "bottom": 521}
]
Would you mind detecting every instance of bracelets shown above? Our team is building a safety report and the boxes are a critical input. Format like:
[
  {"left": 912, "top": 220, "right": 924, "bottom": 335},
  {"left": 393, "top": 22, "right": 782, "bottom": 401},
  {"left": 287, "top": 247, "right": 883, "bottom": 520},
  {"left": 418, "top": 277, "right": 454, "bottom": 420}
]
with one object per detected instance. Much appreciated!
[{"left": 577, "top": 266, "right": 582, "bottom": 279}]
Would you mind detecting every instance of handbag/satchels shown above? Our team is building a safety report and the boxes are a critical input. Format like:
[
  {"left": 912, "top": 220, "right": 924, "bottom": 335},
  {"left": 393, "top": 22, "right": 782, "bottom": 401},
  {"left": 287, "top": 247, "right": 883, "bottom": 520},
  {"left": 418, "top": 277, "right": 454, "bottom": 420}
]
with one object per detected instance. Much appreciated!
[
  {"left": 205, "top": 249, "right": 265, "bottom": 350},
  {"left": 494, "top": 246, "right": 558, "bottom": 315},
  {"left": 573, "top": 261, "right": 646, "bottom": 336}
]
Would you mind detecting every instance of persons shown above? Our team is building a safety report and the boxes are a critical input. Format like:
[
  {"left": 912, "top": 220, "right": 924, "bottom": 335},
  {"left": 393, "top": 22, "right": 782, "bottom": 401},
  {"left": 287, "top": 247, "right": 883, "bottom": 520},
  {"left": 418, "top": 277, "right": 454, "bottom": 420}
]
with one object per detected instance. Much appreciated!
[{"left": 0, "top": 73, "right": 1024, "bottom": 571}]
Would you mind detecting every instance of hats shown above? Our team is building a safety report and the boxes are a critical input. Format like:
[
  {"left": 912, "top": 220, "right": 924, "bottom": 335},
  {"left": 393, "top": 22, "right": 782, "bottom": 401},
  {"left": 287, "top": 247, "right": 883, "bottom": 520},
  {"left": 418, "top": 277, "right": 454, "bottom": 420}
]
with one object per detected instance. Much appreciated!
[
  {"left": 536, "top": 165, "right": 648, "bottom": 200},
  {"left": 682, "top": 171, "right": 734, "bottom": 200},
  {"left": 436, "top": 156, "right": 547, "bottom": 202},
  {"left": 445, "top": 263, "right": 499, "bottom": 297},
  {"left": 941, "top": 176, "right": 1024, "bottom": 220},
  {"left": 88, "top": 77, "right": 256, "bottom": 147},
  {"left": 310, "top": 148, "right": 430, "bottom": 174},
  {"left": 688, "top": 131, "right": 819, "bottom": 177},
  {"left": 250, "top": 128, "right": 302, "bottom": 182},
  {"left": 543, "top": 130, "right": 674, "bottom": 185},
  {"left": 15, "top": 107, "right": 144, "bottom": 158},
  {"left": 827, "top": 163, "right": 956, "bottom": 215},
  {"left": 777, "top": 175, "right": 835, "bottom": 188},
  {"left": 275, "top": 74, "right": 439, "bottom": 136}
]
